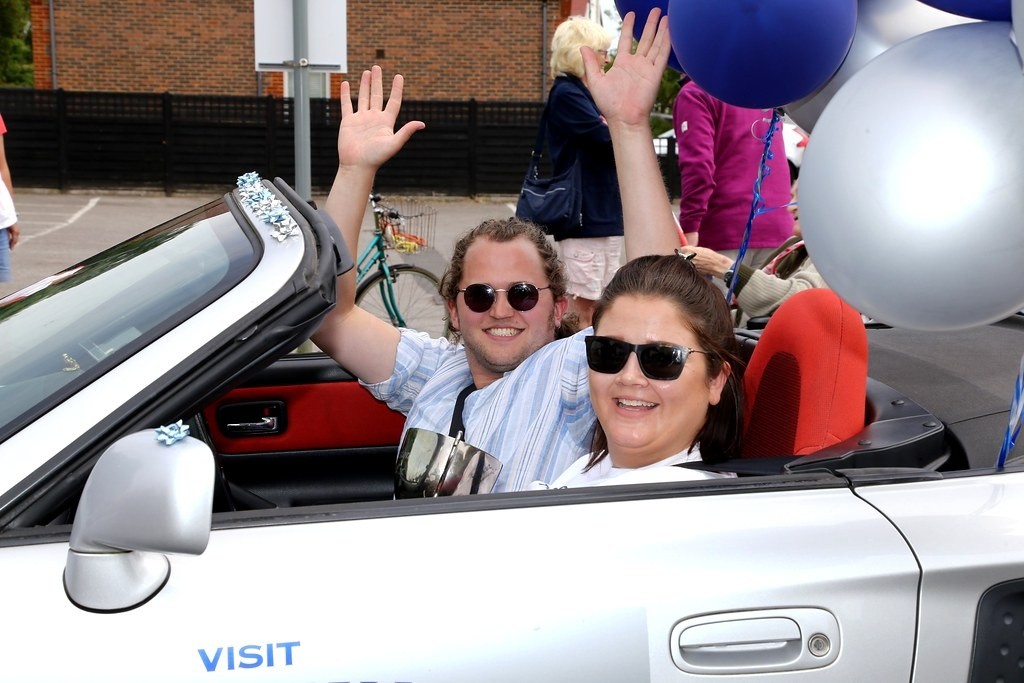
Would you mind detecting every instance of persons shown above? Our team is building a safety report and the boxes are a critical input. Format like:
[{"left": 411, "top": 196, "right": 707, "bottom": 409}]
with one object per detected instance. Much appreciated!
[
  {"left": 546, "top": 256, "right": 750, "bottom": 493},
  {"left": 534, "top": 15, "right": 628, "bottom": 331},
  {"left": 0, "top": 109, "right": 20, "bottom": 282},
  {"left": 308, "top": 8, "right": 679, "bottom": 500},
  {"left": 673, "top": 73, "right": 795, "bottom": 299},
  {"left": 677, "top": 178, "right": 830, "bottom": 331}
]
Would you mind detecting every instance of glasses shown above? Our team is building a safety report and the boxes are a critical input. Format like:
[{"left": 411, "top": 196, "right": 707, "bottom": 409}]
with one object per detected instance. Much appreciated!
[
  {"left": 598, "top": 50, "right": 608, "bottom": 59},
  {"left": 458, "top": 282, "right": 551, "bottom": 313},
  {"left": 585, "top": 336, "right": 721, "bottom": 381}
]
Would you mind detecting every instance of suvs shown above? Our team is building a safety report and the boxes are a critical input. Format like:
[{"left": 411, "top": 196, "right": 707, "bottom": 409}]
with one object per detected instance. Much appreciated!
[{"left": 653, "top": 111, "right": 810, "bottom": 183}]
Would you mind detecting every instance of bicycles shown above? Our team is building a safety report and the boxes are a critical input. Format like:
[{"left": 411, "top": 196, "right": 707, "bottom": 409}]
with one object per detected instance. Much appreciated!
[{"left": 304, "top": 193, "right": 451, "bottom": 341}]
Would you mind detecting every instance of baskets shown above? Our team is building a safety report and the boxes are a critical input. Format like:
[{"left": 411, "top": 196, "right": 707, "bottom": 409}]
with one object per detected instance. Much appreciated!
[{"left": 380, "top": 198, "right": 438, "bottom": 255}]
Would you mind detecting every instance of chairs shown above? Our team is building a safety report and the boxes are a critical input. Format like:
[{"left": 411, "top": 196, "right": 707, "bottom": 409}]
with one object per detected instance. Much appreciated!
[{"left": 743, "top": 287, "right": 871, "bottom": 456}]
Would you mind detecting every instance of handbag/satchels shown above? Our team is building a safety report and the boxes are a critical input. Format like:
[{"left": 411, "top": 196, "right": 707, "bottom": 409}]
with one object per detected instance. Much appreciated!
[{"left": 516, "top": 167, "right": 581, "bottom": 229}]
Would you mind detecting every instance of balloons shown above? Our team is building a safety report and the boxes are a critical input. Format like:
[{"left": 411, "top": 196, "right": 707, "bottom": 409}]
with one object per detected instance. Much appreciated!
[
  {"left": 615, "top": 0, "right": 685, "bottom": 74},
  {"left": 797, "top": 21, "right": 1024, "bottom": 331},
  {"left": 667, "top": 0, "right": 858, "bottom": 109},
  {"left": 780, "top": 0, "right": 986, "bottom": 134},
  {"left": 922, "top": 1, "right": 1013, "bottom": 22},
  {"left": 1011, "top": 1, "right": 1024, "bottom": 62}
]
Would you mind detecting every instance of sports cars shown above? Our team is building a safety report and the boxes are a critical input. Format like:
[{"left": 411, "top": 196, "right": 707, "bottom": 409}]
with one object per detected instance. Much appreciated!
[{"left": 0, "top": 172, "right": 1024, "bottom": 683}]
